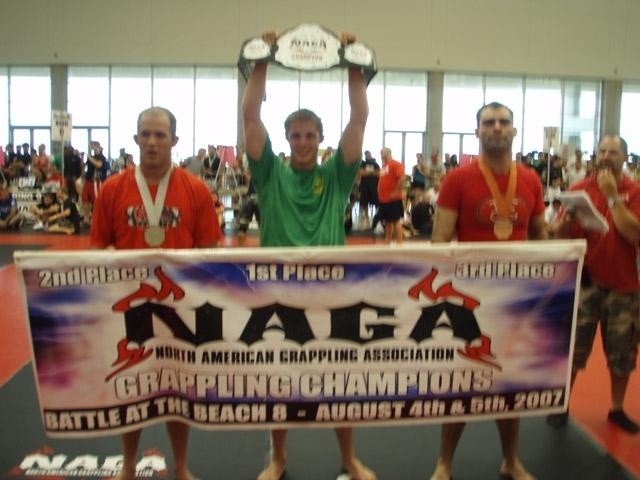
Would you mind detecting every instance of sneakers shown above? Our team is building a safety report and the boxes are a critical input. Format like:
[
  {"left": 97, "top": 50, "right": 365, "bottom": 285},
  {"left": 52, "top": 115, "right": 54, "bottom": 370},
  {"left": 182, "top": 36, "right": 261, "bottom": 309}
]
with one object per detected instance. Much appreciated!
[
  {"left": 547, "top": 408, "right": 569, "bottom": 428},
  {"left": 608, "top": 409, "right": 637, "bottom": 432}
]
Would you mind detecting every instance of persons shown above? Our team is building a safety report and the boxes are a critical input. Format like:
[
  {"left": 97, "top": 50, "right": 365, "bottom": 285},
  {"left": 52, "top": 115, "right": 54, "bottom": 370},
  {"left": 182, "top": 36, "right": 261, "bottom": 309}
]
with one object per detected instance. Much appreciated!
[
  {"left": 546, "top": 134, "right": 640, "bottom": 433},
  {"left": 89, "top": 107, "right": 224, "bottom": 480},
  {"left": 430, "top": 102, "right": 548, "bottom": 480},
  {"left": 621, "top": 155, "right": 640, "bottom": 184},
  {"left": 515, "top": 150, "right": 591, "bottom": 238},
  {"left": 180, "top": 145, "right": 262, "bottom": 235},
  {"left": 242, "top": 32, "right": 377, "bottom": 480},
  {"left": 320, "top": 145, "right": 460, "bottom": 244},
  {"left": 0, "top": 140, "right": 135, "bottom": 234}
]
